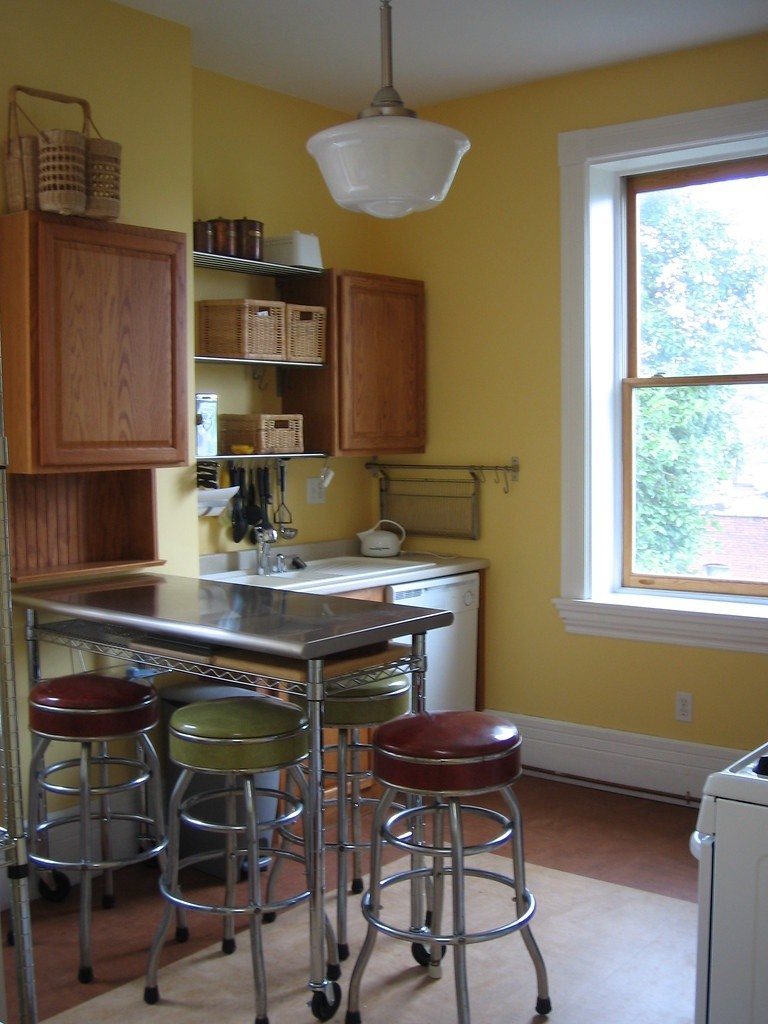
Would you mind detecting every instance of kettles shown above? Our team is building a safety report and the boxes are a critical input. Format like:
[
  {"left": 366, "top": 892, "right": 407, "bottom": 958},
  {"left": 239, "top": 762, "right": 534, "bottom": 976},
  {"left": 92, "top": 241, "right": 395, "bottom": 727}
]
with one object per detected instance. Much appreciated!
[{"left": 356, "top": 520, "right": 406, "bottom": 557}]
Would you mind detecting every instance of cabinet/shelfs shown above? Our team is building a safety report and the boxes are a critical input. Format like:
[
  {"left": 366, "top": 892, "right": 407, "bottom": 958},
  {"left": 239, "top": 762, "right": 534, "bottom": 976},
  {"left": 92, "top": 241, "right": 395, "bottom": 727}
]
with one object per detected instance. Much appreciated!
[
  {"left": 276, "top": 571, "right": 482, "bottom": 821},
  {"left": 1, "top": 208, "right": 191, "bottom": 475},
  {"left": 284, "top": 269, "right": 426, "bottom": 456},
  {"left": 689, "top": 794, "right": 768, "bottom": 1024}
]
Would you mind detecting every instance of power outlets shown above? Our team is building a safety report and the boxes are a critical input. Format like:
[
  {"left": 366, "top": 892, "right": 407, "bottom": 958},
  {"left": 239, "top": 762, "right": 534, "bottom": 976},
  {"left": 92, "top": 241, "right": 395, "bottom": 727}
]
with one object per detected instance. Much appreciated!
[{"left": 675, "top": 693, "right": 692, "bottom": 722}]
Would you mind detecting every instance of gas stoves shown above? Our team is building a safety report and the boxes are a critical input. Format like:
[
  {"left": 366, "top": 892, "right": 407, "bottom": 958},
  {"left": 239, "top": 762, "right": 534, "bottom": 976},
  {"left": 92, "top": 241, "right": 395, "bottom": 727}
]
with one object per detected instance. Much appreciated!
[{"left": 703, "top": 741, "right": 768, "bottom": 806}]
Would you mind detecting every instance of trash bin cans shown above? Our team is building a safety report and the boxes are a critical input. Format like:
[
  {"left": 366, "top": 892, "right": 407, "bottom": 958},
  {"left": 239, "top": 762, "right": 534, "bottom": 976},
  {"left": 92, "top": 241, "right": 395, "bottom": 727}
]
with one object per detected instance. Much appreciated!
[{"left": 157, "top": 686, "right": 281, "bottom": 881}]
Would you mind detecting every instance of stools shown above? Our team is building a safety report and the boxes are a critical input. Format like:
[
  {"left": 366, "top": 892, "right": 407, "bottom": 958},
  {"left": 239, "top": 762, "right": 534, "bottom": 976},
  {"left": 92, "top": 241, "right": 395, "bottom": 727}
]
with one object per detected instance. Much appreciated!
[
  {"left": 264, "top": 667, "right": 435, "bottom": 927},
  {"left": 143, "top": 697, "right": 340, "bottom": 1024},
  {"left": 7, "top": 674, "right": 190, "bottom": 984},
  {"left": 344, "top": 709, "right": 552, "bottom": 1024}
]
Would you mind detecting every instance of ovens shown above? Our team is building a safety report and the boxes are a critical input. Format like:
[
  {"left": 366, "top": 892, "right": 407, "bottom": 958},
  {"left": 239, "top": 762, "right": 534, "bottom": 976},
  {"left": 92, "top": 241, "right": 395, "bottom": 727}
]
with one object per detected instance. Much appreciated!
[{"left": 690, "top": 796, "right": 768, "bottom": 1024}]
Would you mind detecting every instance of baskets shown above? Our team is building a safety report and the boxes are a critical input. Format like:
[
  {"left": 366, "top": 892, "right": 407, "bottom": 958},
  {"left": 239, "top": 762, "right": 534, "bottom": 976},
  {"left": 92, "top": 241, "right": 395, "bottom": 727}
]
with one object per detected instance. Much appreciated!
[
  {"left": 285, "top": 303, "right": 328, "bottom": 364},
  {"left": 217, "top": 413, "right": 304, "bottom": 455},
  {"left": 0, "top": 85, "right": 122, "bottom": 220},
  {"left": 194, "top": 299, "right": 287, "bottom": 361}
]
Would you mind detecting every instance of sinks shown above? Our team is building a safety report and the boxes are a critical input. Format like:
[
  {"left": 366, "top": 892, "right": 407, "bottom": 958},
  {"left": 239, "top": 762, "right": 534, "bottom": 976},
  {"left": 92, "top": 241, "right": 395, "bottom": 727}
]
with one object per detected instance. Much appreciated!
[
  {"left": 199, "top": 575, "right": 313, "bottom": 590},
  {"left": 268, "top": 569, "right": 349, "bottom": 581}
]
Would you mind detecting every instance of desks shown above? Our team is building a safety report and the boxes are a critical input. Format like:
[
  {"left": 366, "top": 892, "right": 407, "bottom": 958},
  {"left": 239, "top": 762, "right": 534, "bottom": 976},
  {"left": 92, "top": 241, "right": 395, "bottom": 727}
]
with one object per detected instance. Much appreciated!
[{"left": 6, "top": 571, "right": 455, "bottom": 1022}]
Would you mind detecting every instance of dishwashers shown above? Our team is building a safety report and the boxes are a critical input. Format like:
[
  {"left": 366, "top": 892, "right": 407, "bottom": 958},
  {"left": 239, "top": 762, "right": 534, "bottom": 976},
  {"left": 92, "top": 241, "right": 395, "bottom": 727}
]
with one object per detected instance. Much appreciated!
[{"left": 385, "top": 573, "right": 480, "bottom": 712}]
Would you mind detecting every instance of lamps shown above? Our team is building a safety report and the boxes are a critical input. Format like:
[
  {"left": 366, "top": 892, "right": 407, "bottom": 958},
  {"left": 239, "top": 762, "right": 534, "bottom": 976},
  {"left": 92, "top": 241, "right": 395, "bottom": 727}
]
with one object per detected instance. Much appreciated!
[{"left": 306, "top": 0, "right": 471, "bottom": 218}]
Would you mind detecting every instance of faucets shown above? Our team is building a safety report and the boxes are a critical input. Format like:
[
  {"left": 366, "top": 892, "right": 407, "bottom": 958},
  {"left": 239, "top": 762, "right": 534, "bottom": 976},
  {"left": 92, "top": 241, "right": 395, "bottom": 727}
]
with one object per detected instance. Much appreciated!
[
  {"left": 254, "top": 527, "right": 277, "bottom": 575},
  {"left": 276, "top": 554, "right": 286, "bottom": 573}
]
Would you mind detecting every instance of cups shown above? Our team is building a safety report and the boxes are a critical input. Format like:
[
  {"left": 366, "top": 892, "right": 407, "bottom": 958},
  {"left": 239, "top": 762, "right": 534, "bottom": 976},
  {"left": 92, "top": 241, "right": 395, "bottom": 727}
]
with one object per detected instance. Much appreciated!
[
  {"left": 193, "top": 219, "right": 214, "bottom": 254},
  {"left": 210, "top": 217, "right": 237, "bottom": 257},
  {"left": 237, "top": 216, "right": 264, "bottom": 260}
]
